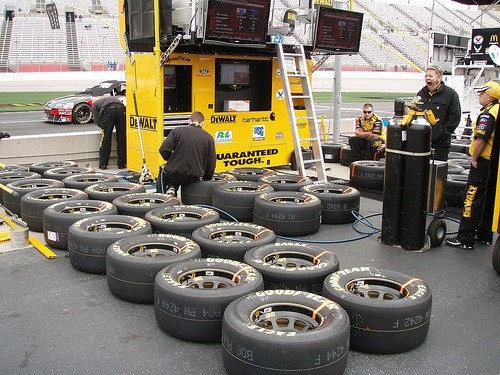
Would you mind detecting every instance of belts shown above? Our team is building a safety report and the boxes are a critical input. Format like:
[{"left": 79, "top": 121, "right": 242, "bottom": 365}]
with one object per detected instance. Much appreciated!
[{"left": 110, "top": 104, "right": 123, "bottom": 107}]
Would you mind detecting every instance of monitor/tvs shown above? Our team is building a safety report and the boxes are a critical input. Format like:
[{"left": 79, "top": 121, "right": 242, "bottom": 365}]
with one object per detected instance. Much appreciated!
[
  {"left": 313, "top": 6, "right": 364, "bottom": 53},
  {"left": 163, "top": 65, "right": 177, "bottom": 89},
  {"left": 202, "top": 0, "right": 271, "bottom": 48},
  {"left": 214, "top": 60, "right": 252, "bottom": 90}
]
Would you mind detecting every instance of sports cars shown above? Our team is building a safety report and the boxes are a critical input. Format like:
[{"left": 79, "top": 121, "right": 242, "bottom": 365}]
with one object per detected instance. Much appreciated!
[{"left": 41, "top": 79, "right": 126, "bottom": 124}]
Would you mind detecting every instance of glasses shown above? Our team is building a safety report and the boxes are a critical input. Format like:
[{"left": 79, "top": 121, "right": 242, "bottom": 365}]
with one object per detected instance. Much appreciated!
[{"left": 363, "top": 110, "right": 372, "bottom": 113}]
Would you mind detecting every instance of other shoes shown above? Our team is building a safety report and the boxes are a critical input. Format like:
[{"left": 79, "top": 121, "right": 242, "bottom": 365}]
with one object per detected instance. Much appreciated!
[
  {"left": 118, "top": 164, "right": 126, "bottom": 168},
  {"left": 100, "top": 164, "right": 106, "bottom": 169},
  {"left": 474, "top": 232, "right": 492, "bottom": 244},
  {"left": 445, "top": 236, "right": 474, "bottom": 249},
  {"left": 166, "top": 184, "right": 175, "bottom": 195}
]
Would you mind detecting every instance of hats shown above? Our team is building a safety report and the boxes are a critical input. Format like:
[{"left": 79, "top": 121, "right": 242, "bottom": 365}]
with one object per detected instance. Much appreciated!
[{"left": 473, "top": 80, "right": 500, "bottom": 99}]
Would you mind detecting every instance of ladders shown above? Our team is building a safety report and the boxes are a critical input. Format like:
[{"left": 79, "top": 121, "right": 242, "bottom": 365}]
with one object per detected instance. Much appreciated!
[{"left": 278, "top": 43, "right": 326, "bottom": 180}]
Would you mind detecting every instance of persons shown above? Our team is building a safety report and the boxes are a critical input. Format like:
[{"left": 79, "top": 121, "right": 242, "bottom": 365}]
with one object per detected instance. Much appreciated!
[
  {"left": 349, "top": 104, "right": 387, "bottom": 161},
  {"left": 156, "top": 112, "right": 216, "bottom": 197},
  {"left": 92, "top": 96, "right": 127, "bottom": 171},
  {"left": 108, "top": 62, "right": 119, "bottom": 70},
  {"left": 417, "top": 64, "right": 461, "bottom": 162},
  {"left": 444, "top": 81, "right": 500, "bottom": 249}
]
